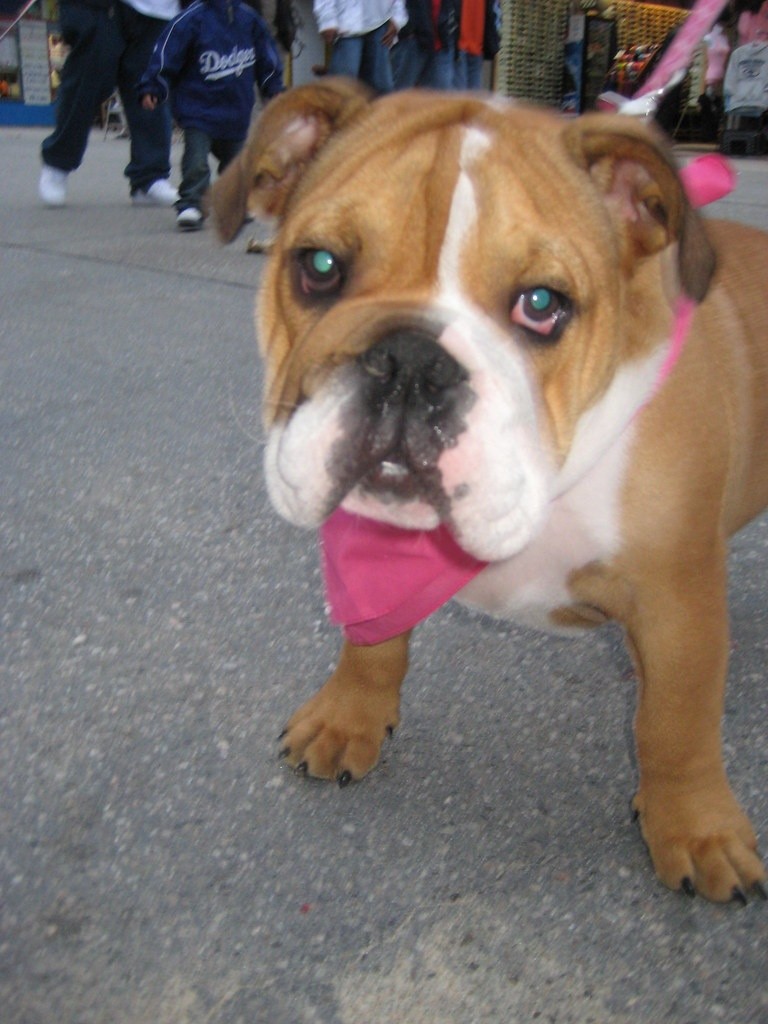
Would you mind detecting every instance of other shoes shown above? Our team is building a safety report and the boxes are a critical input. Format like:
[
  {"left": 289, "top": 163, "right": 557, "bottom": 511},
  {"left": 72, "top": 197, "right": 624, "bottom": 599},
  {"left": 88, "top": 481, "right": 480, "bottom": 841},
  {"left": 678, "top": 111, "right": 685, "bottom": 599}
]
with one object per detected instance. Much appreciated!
[{"left": 177, "top": 209, "right": 203, "bottom": 228}]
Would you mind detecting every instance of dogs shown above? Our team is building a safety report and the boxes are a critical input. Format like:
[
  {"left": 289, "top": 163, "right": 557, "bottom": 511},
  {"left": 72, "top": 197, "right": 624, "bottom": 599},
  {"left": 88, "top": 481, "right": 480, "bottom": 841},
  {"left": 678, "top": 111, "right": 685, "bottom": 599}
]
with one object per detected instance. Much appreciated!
[{"left": 202, "top": 73, "right": 767, "bottom": 909}]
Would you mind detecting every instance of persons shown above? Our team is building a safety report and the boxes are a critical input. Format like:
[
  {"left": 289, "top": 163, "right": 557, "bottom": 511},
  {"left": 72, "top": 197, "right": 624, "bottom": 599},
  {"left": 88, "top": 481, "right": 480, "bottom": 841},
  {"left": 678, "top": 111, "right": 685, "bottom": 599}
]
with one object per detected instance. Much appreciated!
[
  {"left": 702, "top": 24, "right": 731, "bottom": 94},
  {"left": 36, "top": 1, "right": 508, "bottom": 227}
]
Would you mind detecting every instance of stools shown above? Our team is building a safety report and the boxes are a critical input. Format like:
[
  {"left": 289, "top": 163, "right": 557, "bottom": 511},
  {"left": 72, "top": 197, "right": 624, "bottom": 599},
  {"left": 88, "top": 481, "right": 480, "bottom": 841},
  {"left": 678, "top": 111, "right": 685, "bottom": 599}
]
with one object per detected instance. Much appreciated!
[{"left": 719, "top": 127, "right": 761, "bottom": 155}]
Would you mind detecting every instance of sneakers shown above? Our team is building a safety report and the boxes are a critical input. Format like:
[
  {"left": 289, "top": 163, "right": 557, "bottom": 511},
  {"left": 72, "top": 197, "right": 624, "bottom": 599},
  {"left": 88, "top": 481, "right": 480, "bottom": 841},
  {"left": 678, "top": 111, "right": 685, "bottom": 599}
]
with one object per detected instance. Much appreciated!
[
  {"left": 132, "top": 178, "right": 181, "bottom": 205},
  {"left": 38, "top": 160, "right": 70, "bottom": 207}
]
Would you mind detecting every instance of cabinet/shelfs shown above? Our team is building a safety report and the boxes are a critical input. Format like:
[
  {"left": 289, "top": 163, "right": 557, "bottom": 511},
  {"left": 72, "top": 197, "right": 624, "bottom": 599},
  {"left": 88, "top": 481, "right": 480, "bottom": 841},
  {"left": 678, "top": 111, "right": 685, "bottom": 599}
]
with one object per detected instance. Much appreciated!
[{"left": 558, "top": 13, "right": 617, "bottom": 118}]
